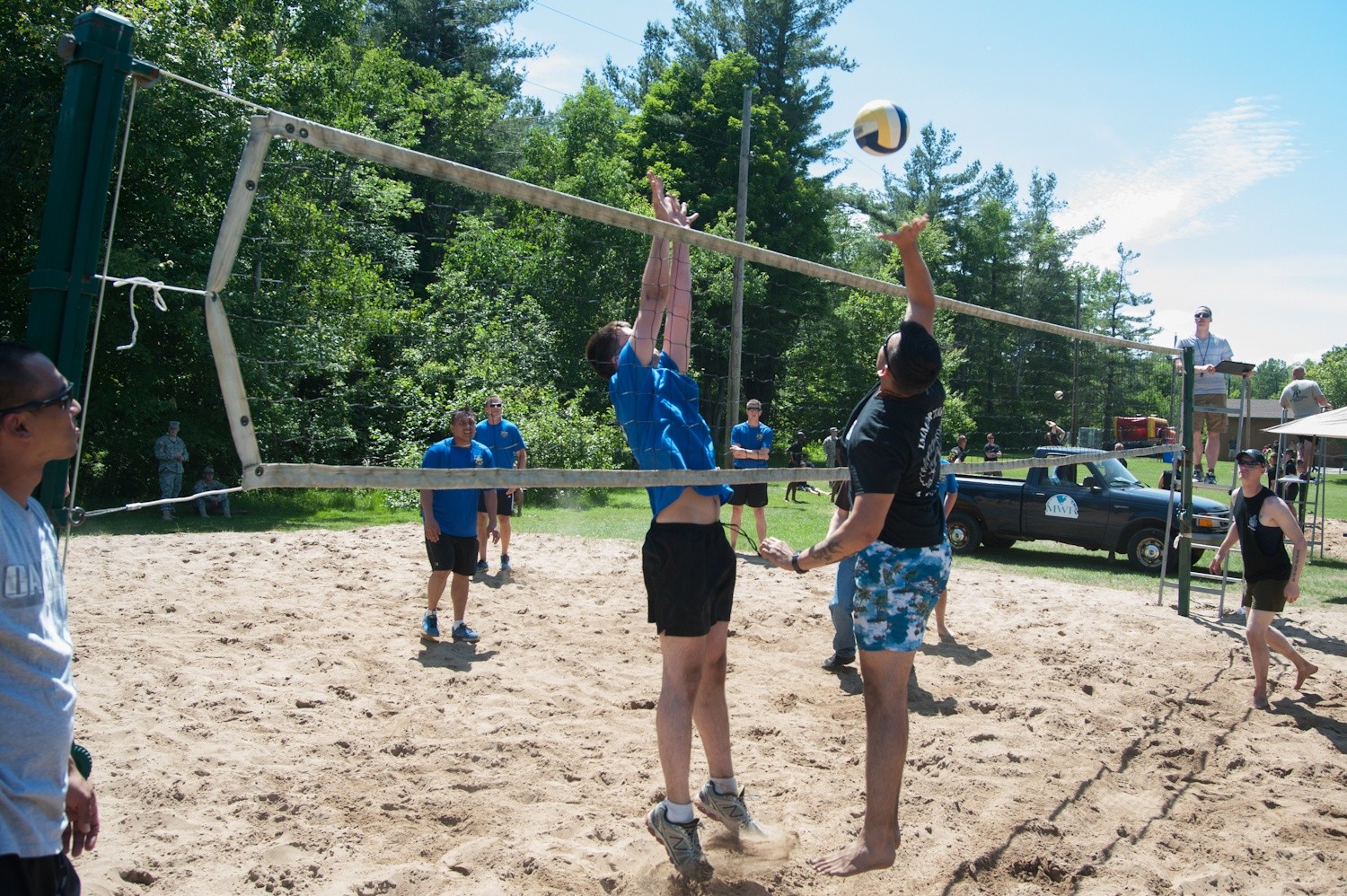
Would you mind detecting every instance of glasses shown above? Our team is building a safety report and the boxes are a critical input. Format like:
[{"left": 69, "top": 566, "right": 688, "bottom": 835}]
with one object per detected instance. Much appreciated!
[
  {"left": 883, "top": 331, "right": 902, "bottom": 382},
  {"left": 1236, "top": 459, "right": 1260, "bottom": 468},
  {"left": 1194, "top": 313, "right": 1210, "bottom": 318},
  {"left": 487, "top": 403, "right": 503, "bottom": 407},
  {"left": 0, "top": 382, "right": 76, "bottom": 414},
  {"left": 746, "top": 407, "right": 761, "bottom": 412},
  {"left": 989, "top": 437, "right": 995, "bottom": 439}
]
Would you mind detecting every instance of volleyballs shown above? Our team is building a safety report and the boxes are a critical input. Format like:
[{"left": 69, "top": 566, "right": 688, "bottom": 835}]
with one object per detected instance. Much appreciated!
[
  {"left": 853, "top": 99, "right": 910, "bottom": 158},
  {"left": 1053, "top": 390, "right": 1063, "bottom": 400}
]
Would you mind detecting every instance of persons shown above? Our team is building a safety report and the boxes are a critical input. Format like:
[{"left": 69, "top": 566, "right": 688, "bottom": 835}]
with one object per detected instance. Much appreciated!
[
  {"left": 1172, "top": 305, "right": 1234, "bottom": 484},
  {"left": 783, "top": 427, "right": 840, "bottom": 504},
  {"left": 933, "top": 440, "right": 958, "bottom": 638},
  {"left": 466, "top": 395, "right": 526, "bottom": 573},
  {"left": 0, "top": 343, "right": 100, "bottom": 896},
  {"left": 1209, "top": 449, "right": 1319, "bottom": 710},
  {"left": 416, "top": 410, "right": 500, "bottom": 642},
  {"left": 153, "top": 421, "right": 189, "bottom": 521},
  {"left": 194, "top": 467, "right": 232, "bottom": 519},
  {"left": 584, "top": 166, "right": 761, "bottom": 884},
  {"left": 759, "top": 211, "right": 952, "bottom": 880},
  {"left": 727, "top": 399, "right": 772, "bottom": 557},
  {"left": 947, "top": 421, "right": 1302, "bottom": 541},
  {"left": 1278, "top": 367, "right": 1333, "bottom": 480},
  {"left": 822, "top": 466, "right": 862, "bottom": 670}
]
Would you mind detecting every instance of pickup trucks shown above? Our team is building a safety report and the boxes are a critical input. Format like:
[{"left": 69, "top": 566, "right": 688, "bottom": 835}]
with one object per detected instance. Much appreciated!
[{"left": 943, "top": 444, "right": 1234, "bottom": 574}]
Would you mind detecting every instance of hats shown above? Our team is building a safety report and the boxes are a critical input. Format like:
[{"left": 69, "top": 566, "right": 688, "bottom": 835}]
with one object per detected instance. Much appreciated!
[
  {"left": 203, "top": 467, "right": 214, "bottom": 474},
  {"left": 169, "top": 421, "right": 180, "bottom": 428},
  {"left": 1234, "top": 448, "right": 1266, "bottom": 468}
]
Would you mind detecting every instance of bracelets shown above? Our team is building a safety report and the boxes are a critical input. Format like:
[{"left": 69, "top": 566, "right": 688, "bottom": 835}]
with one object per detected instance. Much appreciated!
[{"left": 791, "top": 551, "right": 809, "bottom": 574}]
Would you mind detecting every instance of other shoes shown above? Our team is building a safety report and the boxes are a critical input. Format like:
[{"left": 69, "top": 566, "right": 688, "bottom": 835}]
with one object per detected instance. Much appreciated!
[
  {"left": 163, "top": 515, "right": 173, "bottom": 520},
  {"left": 223, "top": 515, "right": 234, "bottom": 518},
  {"left": 171, "top": 515, "right": 179, "bottom": 519},
  {"left": 1300, "top": 472, "right": 1315, "bottom": 480},
  {"left": 202, "top": 514, "right": 211, "bottom": 518},
  {"left": 1297, "top": 458, "right": 1305, "bottom": 473}
]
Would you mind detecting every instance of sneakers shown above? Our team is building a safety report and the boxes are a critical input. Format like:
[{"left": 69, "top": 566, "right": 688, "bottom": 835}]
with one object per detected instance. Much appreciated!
[
  {"left": 821, "top": 649, "right": 855, "bottom": 667},
  {"left": 424, "top": 609, "right": 442, "bottom": 637},
  {"left": 694, "top": 780, "right": 772, "bottom": 841},
  {"left": 502, "top": 561, "right": 510, "bottom": 570},
  {"left": 451, "top": 623, "right": 481, "bottom": 641},
  {"left": 476, "top": 561, "right": 488, "bottom": 572},
  {"left": 1204, "top": 473, "right": 1216, "bottom": 484},
  {"left": 1192, "top": 469, "right": 1205, "bottom": 484},
  {"left": 645, "top": 797, "right": 715, "bottom": 881}
]
